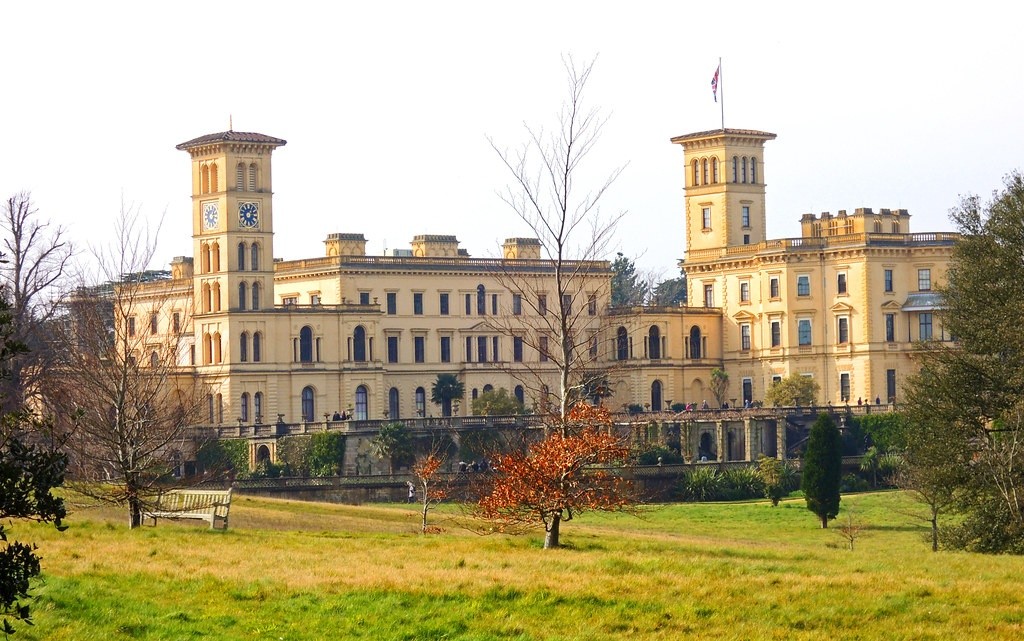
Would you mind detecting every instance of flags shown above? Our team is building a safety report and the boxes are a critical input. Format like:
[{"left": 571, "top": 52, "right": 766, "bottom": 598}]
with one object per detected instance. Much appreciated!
[{"left": 711, "top": 66, "right": 719, "bottom": 102}]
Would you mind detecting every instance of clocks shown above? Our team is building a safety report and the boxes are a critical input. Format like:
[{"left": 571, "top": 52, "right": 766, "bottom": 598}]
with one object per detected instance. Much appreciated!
[
  {"left": 202, "top": 201, "right": 218, "bottom": 230},
  {"left": 238, "top": 202, "right": 259, "bottom": 228}
]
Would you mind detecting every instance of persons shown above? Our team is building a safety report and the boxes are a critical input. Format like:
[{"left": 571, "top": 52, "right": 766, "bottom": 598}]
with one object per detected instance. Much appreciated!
[
  {"left": 686, "top": 402, "right": 691, "bottom": 409},
  {"left": 876, "top": 395, "right": 880, "bottom": 404},
  {"left": 722, "top": 401, "right": 729, "bottom": 409},
  {"left": 333, "top": 411, "right": 346, "bottom": 421},
  {"left": 858, "top": 397, "right": 862, "bottom": 405},
  {"left": 459, "top": 460, "right": 478, "bottom": 472},
  {"left": 480, "top": 459, "right": 488, "bottom": 472},
  {"left": 407, "top": 481, "right": 416, "bottom": 504},
  {"left": 746, "top": 400, "right": 751, "bottom": 407},
  {"left": 703, "top": 400, "right": 709, "bottom": 408}
]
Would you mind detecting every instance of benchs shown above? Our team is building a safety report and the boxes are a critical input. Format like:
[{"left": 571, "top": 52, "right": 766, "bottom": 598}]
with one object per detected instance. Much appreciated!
[{"left": 139, "top": 487, "right": 233, "bottom": 530}]
